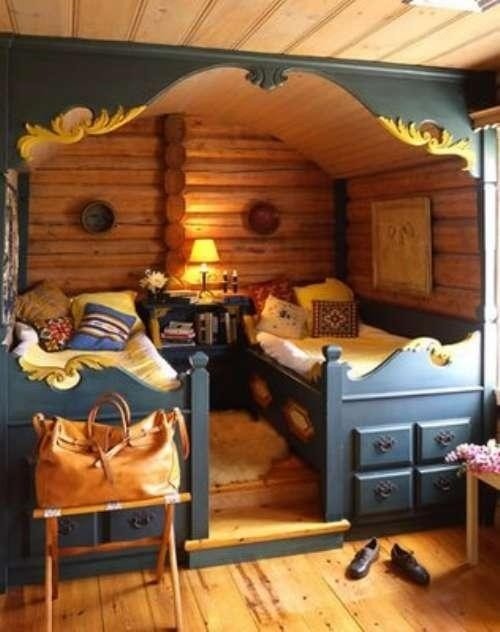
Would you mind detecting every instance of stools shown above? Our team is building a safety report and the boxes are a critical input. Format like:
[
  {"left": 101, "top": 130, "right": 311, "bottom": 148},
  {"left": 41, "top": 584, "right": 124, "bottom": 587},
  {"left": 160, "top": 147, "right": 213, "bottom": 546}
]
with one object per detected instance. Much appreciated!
[
  {"left": 32, "top": 492, "right": 193, "bottom": 632},
  {"left": 465, "top": 458, "right": 499, "bottom": 564}
]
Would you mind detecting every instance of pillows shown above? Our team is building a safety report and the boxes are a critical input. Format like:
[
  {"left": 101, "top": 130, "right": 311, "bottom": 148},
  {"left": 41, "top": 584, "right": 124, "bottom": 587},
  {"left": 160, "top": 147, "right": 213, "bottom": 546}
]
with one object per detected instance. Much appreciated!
[
  {"left": 254, "top": 276, "right": 359, "bottom": 339},
  {"left": 15, "top": 278, "right": 145, "bottom": 351}
]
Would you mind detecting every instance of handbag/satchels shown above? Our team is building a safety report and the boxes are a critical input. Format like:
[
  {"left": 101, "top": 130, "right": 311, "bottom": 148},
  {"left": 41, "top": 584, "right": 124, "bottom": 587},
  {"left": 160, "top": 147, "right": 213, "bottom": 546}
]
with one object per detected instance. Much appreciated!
[{"left": 34, "top": 409, "right": 182, "bottom": 510}]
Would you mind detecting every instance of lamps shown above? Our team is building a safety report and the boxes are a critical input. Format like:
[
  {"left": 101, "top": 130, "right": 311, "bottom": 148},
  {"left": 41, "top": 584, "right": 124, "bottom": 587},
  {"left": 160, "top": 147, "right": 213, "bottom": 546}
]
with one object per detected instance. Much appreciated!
[{"left": 189, "top": 239, "right": 219, "bottom": 290}]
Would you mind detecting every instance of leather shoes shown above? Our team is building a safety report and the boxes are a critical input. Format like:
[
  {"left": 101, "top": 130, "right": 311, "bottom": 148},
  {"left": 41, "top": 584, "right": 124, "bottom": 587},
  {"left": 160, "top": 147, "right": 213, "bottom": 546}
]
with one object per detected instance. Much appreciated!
[
  {"left": 392, "top": 544, "right": 430, "bottom": 584},
  {"left": 350, "top": 538, "right": 379, "bottom": 580}
]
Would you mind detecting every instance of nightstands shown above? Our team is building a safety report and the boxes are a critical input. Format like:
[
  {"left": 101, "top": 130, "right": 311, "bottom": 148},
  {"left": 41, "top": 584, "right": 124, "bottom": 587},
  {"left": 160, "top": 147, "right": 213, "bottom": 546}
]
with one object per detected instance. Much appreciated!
[{"left": 144, "top": 290, "right": 260, "bottom": 422}]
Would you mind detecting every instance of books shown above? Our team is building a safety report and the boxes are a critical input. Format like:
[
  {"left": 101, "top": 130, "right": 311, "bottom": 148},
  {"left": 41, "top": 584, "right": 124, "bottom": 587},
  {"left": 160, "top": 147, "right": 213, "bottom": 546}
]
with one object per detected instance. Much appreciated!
[{"left": 160, "top": 304, "right": 238, "bottom": 347}]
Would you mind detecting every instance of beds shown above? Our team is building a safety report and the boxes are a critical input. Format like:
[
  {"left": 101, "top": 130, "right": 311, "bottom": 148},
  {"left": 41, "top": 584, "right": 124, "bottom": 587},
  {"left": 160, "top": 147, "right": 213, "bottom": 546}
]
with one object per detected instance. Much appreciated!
[
  {"left": 243, "top": 273, "right": 483, "bottom": 523},
  {"left": 0, "top": 278, "right": 210, "bottom": 562}
]
